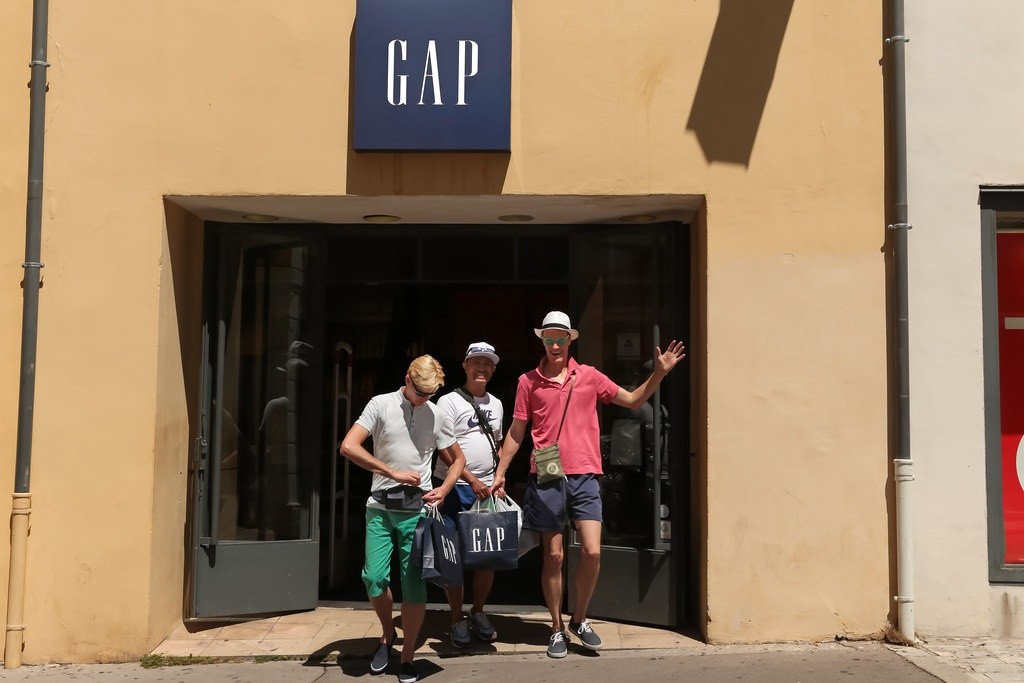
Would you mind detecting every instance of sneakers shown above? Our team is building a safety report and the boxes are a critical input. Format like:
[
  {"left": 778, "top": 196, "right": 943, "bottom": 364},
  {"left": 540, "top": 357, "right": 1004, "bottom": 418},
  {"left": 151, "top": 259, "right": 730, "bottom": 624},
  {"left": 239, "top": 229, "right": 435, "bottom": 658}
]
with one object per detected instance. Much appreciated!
[
  {"left": 451, "top": 615, "right": 471, "bottom": 648},
  {"left": 568, "top": 616, "right": 602, "bottom": 650},
  {"left": 547, "top": 631, "right": 570, "bottom": 657},
  {"left": 467, "top": 607, "right": 497, "bottom": 640}
]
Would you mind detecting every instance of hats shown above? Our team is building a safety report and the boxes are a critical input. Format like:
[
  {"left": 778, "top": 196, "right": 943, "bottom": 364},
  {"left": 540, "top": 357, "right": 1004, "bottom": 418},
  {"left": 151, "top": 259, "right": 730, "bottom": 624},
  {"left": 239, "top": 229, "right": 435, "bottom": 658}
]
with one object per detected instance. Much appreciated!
[
  {"left": 281, "top": 341, "right": 317, "bottom": 363},
  {"left": 534, "top": 311, "right": 579, "bottom": 341},
  {"left": 275, "top": 357, "right": 312, "bottom": 381},
  {"left": 465, "top": 342, "right": 500, "bottom": 364}
]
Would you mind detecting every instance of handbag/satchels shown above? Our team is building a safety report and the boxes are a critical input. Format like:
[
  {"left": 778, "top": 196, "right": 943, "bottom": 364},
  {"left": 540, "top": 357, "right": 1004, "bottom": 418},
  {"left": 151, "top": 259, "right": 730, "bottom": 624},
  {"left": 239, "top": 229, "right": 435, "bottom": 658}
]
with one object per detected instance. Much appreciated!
[
  {"left": 457, "top": 495, "right": 518, "bottom": 571},
  {"left": 410, "top": 503, "right": 462, "bottom": 589},
  {"left": 534, "top": 445, "right": 563, "bottom": 484},
  {"left": 494, "top": 493, "right": 540, "bottom": 558}
]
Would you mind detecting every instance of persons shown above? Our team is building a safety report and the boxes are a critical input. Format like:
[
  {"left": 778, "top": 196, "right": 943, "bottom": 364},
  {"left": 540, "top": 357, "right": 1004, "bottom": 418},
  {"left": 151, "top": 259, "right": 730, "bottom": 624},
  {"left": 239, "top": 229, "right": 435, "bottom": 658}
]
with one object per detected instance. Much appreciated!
[
  {"left": 434, "top": 342, "right": 506, "bottom": 648},
  {"left": 258, "top": 341, "right": 314, "bottom": 466},
  {"left": 340, "top": 355, "right": 466, "bottom": 683},
  {"left": 219, "top": 408, "right": 239, "bottom": 469},
  {"left": 488, "top": 311, "right": 686, "bottom": 658}
]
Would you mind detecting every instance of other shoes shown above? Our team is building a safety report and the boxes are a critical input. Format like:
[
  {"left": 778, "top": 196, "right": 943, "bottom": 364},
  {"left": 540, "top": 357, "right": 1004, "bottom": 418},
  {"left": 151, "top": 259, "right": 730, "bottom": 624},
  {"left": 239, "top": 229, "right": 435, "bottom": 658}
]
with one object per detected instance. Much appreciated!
[
  {"left": 398, "top": 661, "right": 419, "bottom": 683},
  {"left": 370, "top": 626, "right": 398, "bottom": 672}
]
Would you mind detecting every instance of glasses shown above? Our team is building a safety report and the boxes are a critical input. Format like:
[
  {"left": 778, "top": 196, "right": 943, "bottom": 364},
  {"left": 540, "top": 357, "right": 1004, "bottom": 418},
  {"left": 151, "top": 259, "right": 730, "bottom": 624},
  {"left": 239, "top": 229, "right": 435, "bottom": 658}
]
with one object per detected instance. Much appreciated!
[
  {"left": 465, "top": 347, "right": 495, "bottom": 354},
  {"left": 542, "top": 335, "right": 570, "bottom": 346},
  {"left": 407, "top": 376, "right": 437, "bottom": 397}
]
